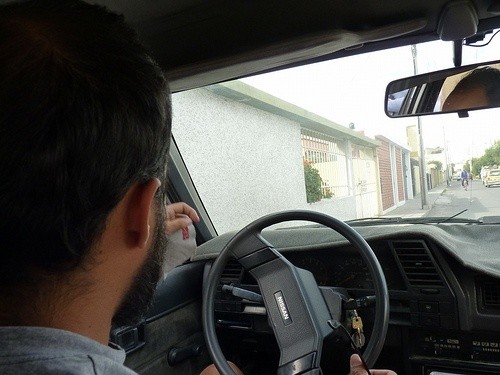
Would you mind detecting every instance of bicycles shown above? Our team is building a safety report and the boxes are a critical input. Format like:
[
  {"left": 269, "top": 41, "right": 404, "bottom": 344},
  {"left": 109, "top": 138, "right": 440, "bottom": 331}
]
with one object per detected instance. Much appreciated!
[{"left": 462, "top": 178, "right": 469, "bottom": 190}]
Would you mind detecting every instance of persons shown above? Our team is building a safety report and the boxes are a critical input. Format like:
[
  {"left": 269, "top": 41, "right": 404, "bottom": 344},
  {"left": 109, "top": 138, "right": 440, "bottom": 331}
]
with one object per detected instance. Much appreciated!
[
  {"left": 443, "top": 67, "right": 500, "bottom": 116},
  {"left": 1, "top": 1, "right": 399, "bottom": 374},
  {"left": 461, "top": 170, "right": 469, "bottom": 187}
]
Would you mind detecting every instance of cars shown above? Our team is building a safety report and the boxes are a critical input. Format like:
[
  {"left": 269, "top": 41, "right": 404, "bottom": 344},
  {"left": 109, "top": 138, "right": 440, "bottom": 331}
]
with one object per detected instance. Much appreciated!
[
  {"left": 484, "top": 169, "right": 500, "bottom": 187},
  {"left": 451, "top": 166, "right": 491, "bottom": 183}
]
